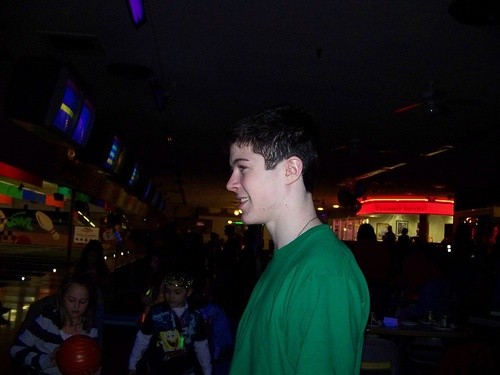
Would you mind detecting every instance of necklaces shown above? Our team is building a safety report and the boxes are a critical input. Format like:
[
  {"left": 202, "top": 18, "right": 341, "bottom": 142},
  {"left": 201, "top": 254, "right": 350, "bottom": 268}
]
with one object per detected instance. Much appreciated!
[{"left": 298, "top": 216, "right": 318, "bottom": 237}]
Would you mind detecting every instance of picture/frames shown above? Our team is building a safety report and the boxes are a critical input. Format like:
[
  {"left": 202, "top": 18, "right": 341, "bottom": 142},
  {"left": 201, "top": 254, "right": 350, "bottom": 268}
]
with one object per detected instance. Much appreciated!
[
  {"left": 396, "top": 221, "right": 408, "bottom": 237},
  {"left": 376, "top": 223, "right": 389, "bottom": 242}
]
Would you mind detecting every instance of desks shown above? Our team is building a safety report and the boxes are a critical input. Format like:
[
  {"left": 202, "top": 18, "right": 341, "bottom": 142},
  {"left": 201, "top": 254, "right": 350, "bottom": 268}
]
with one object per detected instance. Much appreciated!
[{"left": 365, "top": 327, "right": 473, "bottom": 375}]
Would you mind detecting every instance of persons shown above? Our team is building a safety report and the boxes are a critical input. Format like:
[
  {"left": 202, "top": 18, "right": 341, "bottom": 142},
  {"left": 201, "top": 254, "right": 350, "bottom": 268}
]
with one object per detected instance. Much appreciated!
[
  {"left": 225, "top": 104, "right": 371, "bottom": 375},
  {"left": 9, "top": 224, "right": 275, "bottom": 375},
  {"left": 349, "top": 224, "right": 499, "bottom": 325}
]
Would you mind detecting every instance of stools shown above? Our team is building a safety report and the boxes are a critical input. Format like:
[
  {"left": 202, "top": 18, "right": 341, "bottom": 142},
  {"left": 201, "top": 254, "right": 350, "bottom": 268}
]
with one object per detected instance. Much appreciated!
[{"left": 361, "top": 337, "right": 399, "bottom": 374}]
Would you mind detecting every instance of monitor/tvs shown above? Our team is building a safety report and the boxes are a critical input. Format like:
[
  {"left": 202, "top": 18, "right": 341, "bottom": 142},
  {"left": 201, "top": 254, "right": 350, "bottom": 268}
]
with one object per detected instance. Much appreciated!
[{"left": 46, "top": 76, "right": 139, "bottom": 186}]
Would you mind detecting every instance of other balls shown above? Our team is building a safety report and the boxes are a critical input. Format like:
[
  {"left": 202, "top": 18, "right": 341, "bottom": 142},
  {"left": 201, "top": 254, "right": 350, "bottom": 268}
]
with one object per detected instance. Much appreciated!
[{"left": 55, "top": 334, "right": 102, "bottom": 375}]
